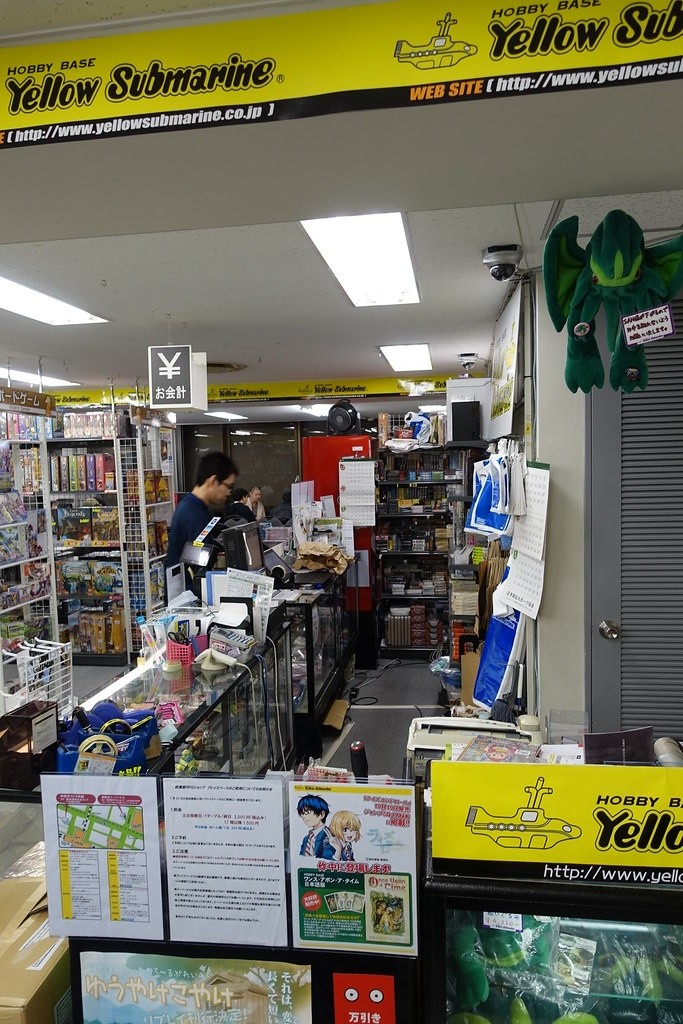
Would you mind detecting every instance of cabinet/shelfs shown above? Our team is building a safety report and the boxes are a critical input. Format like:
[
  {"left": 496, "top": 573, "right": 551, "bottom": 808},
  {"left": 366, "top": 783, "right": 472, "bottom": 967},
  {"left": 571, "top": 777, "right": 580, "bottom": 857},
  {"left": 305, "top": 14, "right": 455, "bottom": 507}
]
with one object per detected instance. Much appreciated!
[
  {"left": 1, "top": 405, "right": 137, "bottom": 666},
  {"left": 449, "top": 564, "right": 480, "bottom": 660},
  {"left": 0, "top": 553, "right": 361, "bottom": 804},
  {"left": 0, "top": 416, "right": 63, "bottom": 715},
  {"left": 374, "top": 447, "right": 467, "bottom": 659},
  {"left": 421, "top": 758, "right": 683, "bottom": 1024},
  {"left": 115, "top": 438, "right": 175, "bottom": 665}
]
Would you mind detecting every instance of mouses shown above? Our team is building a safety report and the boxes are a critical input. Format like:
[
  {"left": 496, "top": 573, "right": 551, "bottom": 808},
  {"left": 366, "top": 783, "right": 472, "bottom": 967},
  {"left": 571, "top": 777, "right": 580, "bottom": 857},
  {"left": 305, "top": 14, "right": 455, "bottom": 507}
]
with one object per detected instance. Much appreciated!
[{"left": 316, "top": 568, "right": 329, "bottom": 573}]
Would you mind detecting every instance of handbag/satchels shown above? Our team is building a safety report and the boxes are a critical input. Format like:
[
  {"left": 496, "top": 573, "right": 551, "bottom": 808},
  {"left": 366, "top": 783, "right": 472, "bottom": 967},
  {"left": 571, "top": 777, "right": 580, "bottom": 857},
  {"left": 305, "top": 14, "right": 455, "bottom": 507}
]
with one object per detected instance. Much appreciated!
[
  {"left": 405, "top": 412, "right": 433, "bottom": 444},
  {"left": 464, "top": 440, "right": 529, "bottom": 539},
  {"left": 472, "top": 555, "right": 528, "bottom": 714}
]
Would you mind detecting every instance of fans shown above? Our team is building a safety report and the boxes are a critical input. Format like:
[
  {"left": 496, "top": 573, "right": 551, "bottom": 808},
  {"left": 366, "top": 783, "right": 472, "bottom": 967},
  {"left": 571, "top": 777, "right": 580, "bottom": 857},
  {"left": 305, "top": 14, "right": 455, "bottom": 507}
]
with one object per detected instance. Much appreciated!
[{"left": 324, "top": 398, "right": 362, "bottom": 437}]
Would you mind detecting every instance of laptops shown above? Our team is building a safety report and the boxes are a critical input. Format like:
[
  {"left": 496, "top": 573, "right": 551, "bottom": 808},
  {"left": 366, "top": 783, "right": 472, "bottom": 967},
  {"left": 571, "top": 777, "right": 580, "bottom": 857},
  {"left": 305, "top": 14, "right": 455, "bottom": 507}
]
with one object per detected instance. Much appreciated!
[{"left": 262, "top": 548, "right": 333, "bottom": 584}]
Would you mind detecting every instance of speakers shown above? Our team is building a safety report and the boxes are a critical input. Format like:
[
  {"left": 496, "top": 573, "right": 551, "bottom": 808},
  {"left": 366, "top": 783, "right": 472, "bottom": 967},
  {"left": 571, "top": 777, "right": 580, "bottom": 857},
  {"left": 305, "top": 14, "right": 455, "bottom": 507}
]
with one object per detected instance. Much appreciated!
[{"left": 452, "top": 401, "right": 482, "bottom": 442}]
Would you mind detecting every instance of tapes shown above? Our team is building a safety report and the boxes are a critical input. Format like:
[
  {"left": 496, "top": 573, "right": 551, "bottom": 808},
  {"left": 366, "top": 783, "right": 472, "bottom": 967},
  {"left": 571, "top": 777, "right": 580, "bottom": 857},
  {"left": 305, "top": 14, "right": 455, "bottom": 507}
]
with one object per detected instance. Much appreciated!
[
  {"left": 78, "top": 735, "right": 118, "bottom": 756},
  {"left": 99, "top": 718, "right": 132, "bottom": 736}
]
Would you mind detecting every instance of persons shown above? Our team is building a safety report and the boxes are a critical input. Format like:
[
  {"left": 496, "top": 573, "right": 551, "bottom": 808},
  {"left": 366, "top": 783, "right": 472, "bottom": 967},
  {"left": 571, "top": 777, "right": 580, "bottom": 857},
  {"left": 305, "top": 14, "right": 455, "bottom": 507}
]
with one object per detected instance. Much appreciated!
[
  {"left": 225, "top": 489, "right": 258, "bottom": 523},
  {"left": 269, "top": 490, "right": 291, "bottom": 525},
  {"left": 164, "top": 450, "right": 241, "bottom": 606},
  {"left": 246, "top": 486, "right": 266, "bottom": 521}
]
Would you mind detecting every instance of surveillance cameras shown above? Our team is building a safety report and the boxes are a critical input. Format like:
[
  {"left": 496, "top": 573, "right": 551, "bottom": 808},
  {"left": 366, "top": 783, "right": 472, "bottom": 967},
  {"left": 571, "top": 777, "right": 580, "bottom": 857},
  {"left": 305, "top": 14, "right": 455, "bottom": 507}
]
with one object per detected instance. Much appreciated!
[{"left": 481, "top": 245, "right": 523, "bottom": 281}]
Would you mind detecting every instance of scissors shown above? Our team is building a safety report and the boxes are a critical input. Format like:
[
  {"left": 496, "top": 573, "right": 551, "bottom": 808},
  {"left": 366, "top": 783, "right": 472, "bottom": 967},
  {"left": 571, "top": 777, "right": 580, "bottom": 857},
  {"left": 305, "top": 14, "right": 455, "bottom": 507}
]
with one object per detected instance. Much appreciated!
[{"left": 168, "top": 632, "right": 185, "bottom": 644}]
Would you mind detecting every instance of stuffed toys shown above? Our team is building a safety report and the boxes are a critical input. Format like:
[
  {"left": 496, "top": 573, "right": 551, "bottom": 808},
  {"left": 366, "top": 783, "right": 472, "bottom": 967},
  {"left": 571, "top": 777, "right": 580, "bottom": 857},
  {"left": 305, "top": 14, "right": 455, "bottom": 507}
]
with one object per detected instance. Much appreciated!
[
  {"left": 542, "top": 210, "right": 683, "bottom": 395},
  {"left": 444, "top": 911, "right": 683, "bottom": 1024}
]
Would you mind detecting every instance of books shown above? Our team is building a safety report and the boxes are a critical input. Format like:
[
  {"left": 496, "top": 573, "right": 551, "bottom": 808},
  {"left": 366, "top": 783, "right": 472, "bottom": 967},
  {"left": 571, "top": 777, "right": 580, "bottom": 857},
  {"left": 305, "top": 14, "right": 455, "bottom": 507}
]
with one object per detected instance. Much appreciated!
[{"left": 441, "top": 734, "right": 543, "bottom": 764}]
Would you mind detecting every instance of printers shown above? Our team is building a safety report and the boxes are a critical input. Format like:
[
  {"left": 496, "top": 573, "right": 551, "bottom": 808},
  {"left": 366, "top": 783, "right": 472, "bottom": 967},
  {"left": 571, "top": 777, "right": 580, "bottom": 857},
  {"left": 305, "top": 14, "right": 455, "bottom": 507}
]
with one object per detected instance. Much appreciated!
[{"left": 406, "top": 718, "right": 532, "bottom": 783}]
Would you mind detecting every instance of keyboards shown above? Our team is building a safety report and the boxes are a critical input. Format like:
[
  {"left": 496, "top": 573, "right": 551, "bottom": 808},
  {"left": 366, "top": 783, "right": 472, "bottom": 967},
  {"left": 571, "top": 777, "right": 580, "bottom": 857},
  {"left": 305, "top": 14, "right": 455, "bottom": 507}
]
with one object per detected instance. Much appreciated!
[{"left": 272, "top": 589, "right": 301, "bottom": 602}]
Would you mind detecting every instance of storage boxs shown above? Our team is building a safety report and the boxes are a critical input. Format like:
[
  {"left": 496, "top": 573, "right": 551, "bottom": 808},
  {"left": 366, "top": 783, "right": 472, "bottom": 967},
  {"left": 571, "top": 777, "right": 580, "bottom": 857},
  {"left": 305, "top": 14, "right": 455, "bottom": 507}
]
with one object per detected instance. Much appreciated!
[
  {"left": 41, "top": 453, "right": 116, "bottom": 492},
  {"left": 315, "top": 518, "right": 342, "bottom": 528},
  {"left": 0, "top": 617, "right": 53, "bottom": 662},
  {"left": 394, "top": 430, "right": 413, "bottom": 439},
  {"left": 410, "top": 604, "right": 443, "bottom": 646},
  {"left": 63, "top": 412, "right": 128, "bottom": 437},
  {"left": 462, "top": 642, "right": 484, "bottom": 707},
  {"left": 452, "top": 619, "right": 476, "bottom": 659},
  {"left": 1, "top": 564, "right": 51, "bottom": 611},
  {"left": 375, "top": 535, "right": 396, "bottom": 540},
  {"left": 392, "top": 570, "right": 450, "bottom": 595},
  {"left": 390, "top": 605, "right": 411, "bottom": 615},
  {"left": 127, "top": 469, "right": 173, "bottom": 504},
  {"left": 312, "top": 532, "right": 342, "bottom": 544},
  {"left": 0, "top": 841, "right": 75, "bottom": 1024},
  {"left": 401, "top": 499, "right": 423, "bottom": 513},
  {"left": 132, "top": 568, "right": 159, "bottom": 610},
  {"left": 127, "top": 520, "right": 170, "bottom": 559},
  {"left": 60, "top": 599, "right": 127, "bottom": 654},
  {"left": 50, "top": 506, "right": 121, "bottom": 542},
  {"left": 434, "top": 524, "right": 454, "bottom": 551},
  {"left": 379, "top": 469, "right": 464, "bottom": 480},
  {"left": 412, "top": 540, "right": 426, "bottom": 551},
  {"left": 378, "top": 412, "right": 391, "bottom": 448},
  {"left": 0, "top": 412, "right": 54, "bottom": 440},
  {"left": 446, "top": 484, "right": 464, "bottom": 511},
  {"left": 57, "top": 561, "right": 123, "bottom": 596}
]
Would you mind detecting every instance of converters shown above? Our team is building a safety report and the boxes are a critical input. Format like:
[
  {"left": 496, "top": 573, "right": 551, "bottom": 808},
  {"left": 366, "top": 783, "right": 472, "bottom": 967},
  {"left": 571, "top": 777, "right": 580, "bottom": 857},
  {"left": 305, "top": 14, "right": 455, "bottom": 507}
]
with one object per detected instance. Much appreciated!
[{"left": 349, "top": 687, "right": 359, "bottom": 698}]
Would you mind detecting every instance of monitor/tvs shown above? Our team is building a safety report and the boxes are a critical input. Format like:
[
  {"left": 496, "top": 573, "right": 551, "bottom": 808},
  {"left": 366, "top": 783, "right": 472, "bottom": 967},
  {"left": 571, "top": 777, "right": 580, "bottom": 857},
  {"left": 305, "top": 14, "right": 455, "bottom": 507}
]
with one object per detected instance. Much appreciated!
[{"left": 222, "top": 521, "right": 265, "bottom": 574}]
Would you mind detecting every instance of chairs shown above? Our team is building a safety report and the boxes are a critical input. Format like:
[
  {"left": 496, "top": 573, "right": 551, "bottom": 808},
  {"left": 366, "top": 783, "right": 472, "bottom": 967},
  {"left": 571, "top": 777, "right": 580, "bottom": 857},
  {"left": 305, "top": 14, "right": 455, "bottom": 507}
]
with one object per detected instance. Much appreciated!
[
  {"left": 267, "top": 516, "right": 290, "bottom": 525},
  {"left": 222, "top": 514, "right": 248, "bottom": 529}
]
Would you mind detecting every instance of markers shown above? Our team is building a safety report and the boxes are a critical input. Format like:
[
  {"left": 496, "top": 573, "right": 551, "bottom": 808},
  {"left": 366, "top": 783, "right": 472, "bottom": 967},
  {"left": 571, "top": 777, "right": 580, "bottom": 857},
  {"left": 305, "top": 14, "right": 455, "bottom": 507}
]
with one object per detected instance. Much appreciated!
[{"left": 74, "top": 707, "right": 93, "bottom": 732}]
